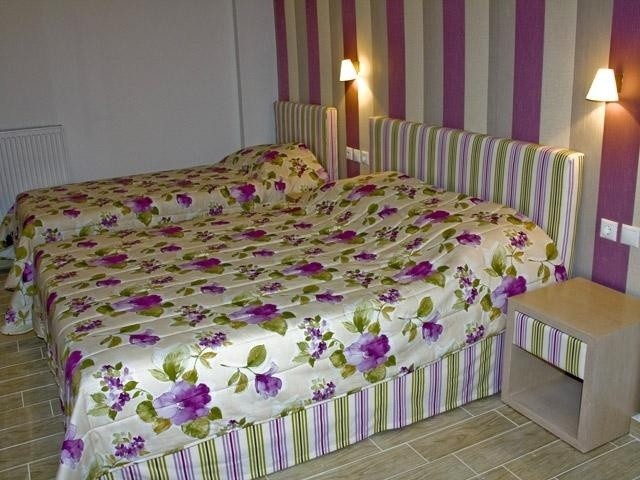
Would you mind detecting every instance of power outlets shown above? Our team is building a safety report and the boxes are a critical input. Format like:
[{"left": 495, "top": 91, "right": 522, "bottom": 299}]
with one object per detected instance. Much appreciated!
[{"left": 599, "top": 217, "right": 619, "bottom": 242}]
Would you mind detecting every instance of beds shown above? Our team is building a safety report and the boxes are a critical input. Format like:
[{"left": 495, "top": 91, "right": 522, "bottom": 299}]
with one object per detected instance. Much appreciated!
[
  {"left": 31, "top": 117, "right": 588, "bottom": 480},
  {"left": 0, "top": 101, "right": 340, "bottom": 334}
]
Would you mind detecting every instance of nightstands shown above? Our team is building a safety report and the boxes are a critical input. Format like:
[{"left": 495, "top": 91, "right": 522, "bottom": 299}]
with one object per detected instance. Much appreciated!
[{"left": 500, "top": 276, "right": 640, "bottom": 454}]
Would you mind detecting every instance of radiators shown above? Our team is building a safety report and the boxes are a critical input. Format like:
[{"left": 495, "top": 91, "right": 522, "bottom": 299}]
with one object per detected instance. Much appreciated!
[{"left": 0, "top": 124, "right": 70, "bottom": 216}]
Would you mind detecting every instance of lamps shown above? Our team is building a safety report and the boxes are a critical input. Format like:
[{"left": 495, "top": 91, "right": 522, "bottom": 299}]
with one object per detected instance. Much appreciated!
[
  {"left": 340, "top": 57, "right": 357, "bottom": 84},
  {"left": 586, "top": 68, "right": 620, "bottom": 104}
]
